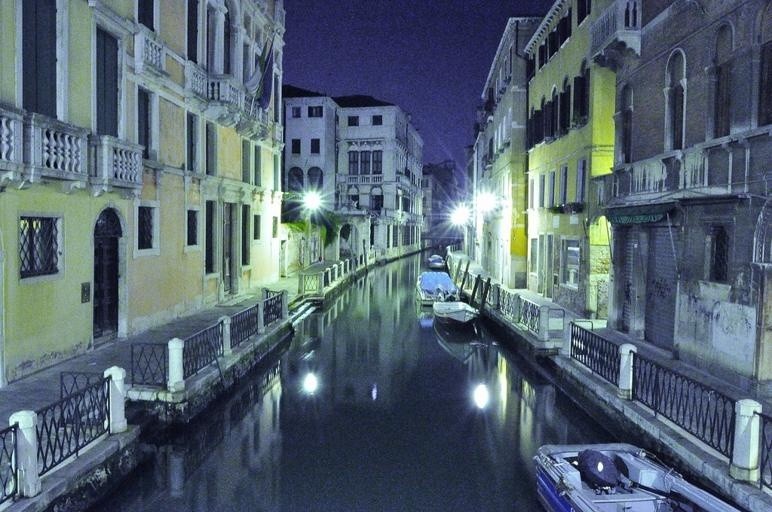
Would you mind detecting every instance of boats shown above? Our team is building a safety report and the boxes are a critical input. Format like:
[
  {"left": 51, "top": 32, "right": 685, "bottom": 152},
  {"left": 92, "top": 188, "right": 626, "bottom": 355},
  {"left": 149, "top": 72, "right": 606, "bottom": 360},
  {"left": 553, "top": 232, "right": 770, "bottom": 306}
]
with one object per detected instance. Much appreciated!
[
  {"left": 416, "top": 271, "right": 459, "bottom": 305},
  {"left": 532, "top": 440, "right": 742, "bottom": 511},
  {"left": 427, "top": 254, "right": 446, "bottom": 268},
  {"left": 433, "top": 300, "right": 481, "bottom": 329}
]
